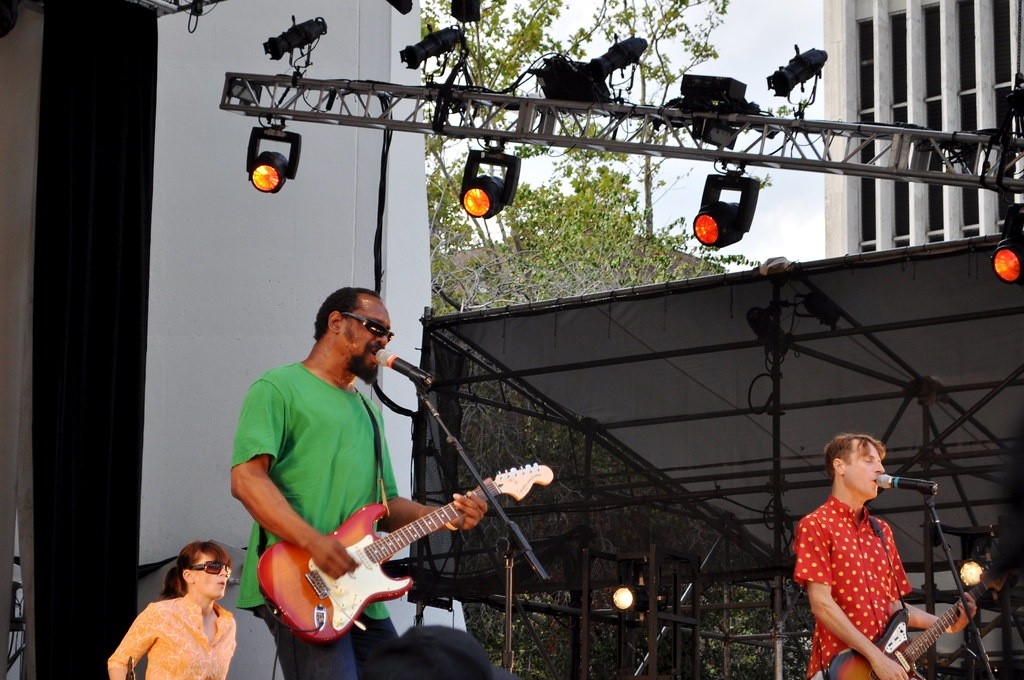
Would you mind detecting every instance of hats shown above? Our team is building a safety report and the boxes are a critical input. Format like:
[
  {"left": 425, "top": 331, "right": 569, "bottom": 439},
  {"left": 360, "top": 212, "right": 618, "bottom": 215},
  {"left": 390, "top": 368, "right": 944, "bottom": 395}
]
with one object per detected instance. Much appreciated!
[{"left": 365, "top": 625, "right": 519, "bottom": 680}]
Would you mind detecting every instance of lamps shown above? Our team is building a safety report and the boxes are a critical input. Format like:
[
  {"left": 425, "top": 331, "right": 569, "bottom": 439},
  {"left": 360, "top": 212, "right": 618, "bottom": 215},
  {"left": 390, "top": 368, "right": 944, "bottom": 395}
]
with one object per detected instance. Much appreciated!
[
  {"left": 400, "top": 25, "right": 464, "bottom": 69},
  {"left": 459, "top": 149, "right": 521, "bottom": 219},
  {"left": 591, "top": 34, "right": 648, "bottom": 78},
  {"left": 767, "top": 43, "right": 828, "bottom": 96},
  {"left": 693, "top": 174, "right": 760, "bottom": 248},
  {"left": 746, "top": 306, "right": 773, "bottom": 339},
  {"left": 805, "top": 291, "right": 841, "bottom": 325},
  {"left": 613, "top": 585, "right": 669, "bottom": 611},
  {"left": 991, "top": 203, "right": 1024, "bottom": 287},
  {"left": 959, "top": 557, "right": 1017, "bottom": 588},
  {"left": 264, "top": 16, "right": 327, "bottom": 60},
  {"left": 246, "top": 127, "right": 302, "bottom": 193}
]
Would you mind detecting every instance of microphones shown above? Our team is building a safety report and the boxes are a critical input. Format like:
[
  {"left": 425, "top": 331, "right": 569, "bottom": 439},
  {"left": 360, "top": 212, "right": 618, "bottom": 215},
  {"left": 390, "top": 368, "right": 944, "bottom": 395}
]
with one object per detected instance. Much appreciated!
[
  {"left": 374, "top": 349, "right": 433, "bottom": 386},
  {"left": 876, "top": 474, "right": 939, "bottom": 495}
]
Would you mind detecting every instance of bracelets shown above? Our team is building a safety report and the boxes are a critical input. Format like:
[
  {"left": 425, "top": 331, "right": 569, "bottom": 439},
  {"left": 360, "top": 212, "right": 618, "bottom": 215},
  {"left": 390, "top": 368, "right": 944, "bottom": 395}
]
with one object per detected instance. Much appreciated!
[{"left": 446, "top": 522, "right": 459, "bottom": 532}]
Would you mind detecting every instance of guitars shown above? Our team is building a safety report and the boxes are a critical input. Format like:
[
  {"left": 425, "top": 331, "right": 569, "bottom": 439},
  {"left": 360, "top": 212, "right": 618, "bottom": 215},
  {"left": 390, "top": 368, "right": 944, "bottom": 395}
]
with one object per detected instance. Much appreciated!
[
  {"left": 257, "top": 460, "right": 555, "bottom": 646},
  {"left": 825, "top": 560, "right": 1009, "bottom": 680}
]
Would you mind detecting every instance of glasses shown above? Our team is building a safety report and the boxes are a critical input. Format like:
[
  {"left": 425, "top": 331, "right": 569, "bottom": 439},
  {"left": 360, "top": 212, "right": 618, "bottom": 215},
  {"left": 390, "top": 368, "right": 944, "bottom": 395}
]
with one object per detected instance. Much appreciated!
[
  {"left": 187, "top": 561, "right": 231, "bottom": 577},
  {"left": 341, "top": 312, "right": 395, "bottom": 344}
]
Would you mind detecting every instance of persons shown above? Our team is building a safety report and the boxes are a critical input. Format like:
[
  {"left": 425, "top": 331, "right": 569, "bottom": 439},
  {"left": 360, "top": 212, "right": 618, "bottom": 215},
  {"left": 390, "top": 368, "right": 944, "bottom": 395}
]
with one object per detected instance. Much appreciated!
[
  {"left": 107, "top": 542, "right": 237, "bottom": 680},
  {"left": 794, "top": 433, "right": 977, "bottom": 680},
  {"left": 231, "top": 286, "right": 488, "bottom": 680},
  {"left": 359, "top": 623, "right": 519, "bottom": 680}
]
